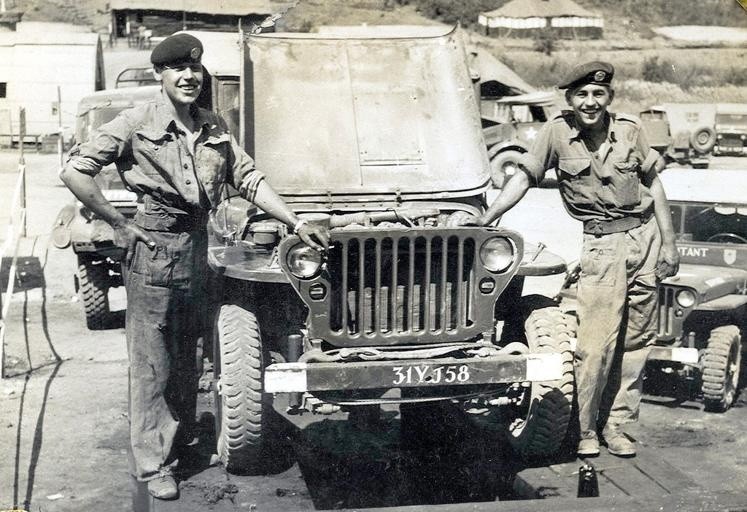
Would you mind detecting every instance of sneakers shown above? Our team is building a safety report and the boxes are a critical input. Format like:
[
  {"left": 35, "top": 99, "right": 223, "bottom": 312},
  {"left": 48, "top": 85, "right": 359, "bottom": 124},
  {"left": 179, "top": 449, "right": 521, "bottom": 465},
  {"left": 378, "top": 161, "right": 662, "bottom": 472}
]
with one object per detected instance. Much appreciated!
[
  {"left": 146, "top": 474, "right": 181, "bottom": 502},
  {"left": 174, "top": 446, "right": 223, "bottom": 472},
  {"left": 596, "top": 418, "right": 638, "bottom": 456},
  {"left": 570, "top": 432, "right": 601, "bottom": 457}
]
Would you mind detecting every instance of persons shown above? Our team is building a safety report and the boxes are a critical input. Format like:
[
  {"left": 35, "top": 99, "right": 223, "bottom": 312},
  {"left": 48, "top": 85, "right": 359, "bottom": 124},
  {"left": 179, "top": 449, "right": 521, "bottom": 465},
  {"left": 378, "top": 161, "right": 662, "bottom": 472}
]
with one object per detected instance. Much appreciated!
[
  {"left": 456, "top": 59, "right": 681, "bottom": 458},
  {"left": 58, "top": 32, "right": 334, "bottom": 501}
]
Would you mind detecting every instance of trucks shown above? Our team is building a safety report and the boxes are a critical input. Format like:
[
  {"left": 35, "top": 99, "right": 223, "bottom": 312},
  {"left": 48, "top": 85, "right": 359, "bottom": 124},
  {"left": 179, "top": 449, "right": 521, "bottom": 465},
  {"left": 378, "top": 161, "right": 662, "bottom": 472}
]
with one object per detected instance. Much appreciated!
[{"left": 483, "top": 92, "right": 670, "bottom": 189}]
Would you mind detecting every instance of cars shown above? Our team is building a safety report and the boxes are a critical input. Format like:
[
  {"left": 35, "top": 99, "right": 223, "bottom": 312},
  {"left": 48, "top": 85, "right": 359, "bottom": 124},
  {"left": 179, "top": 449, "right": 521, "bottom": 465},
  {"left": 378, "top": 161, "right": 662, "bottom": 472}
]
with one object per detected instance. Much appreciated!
[
  {"left": 559, "top": 168, "right": 747, "bottom": 413},
  {"left": 712, "top": 103, "right": 747, "bottom": 156}
]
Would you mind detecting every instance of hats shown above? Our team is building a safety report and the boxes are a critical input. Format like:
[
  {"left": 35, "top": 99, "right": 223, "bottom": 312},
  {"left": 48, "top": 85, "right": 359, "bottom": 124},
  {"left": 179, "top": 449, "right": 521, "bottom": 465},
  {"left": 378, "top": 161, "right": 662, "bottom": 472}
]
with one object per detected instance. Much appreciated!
[
  {"left": 557, "top": 60, "right": 615, "bottom": 91},
  {"left": 149, "top": 33, "right": 204, "bottom": 69}
]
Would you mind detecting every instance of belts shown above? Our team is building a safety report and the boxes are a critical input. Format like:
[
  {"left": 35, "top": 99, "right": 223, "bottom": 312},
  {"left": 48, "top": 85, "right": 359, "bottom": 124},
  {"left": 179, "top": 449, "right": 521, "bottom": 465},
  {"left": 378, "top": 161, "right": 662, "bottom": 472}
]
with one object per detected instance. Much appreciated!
[
  {"left": 583, "top": 213, "right": 644, "bottom": 236},
  {"left": 132, "top": 209, "right": 210, "bottom": 235}
]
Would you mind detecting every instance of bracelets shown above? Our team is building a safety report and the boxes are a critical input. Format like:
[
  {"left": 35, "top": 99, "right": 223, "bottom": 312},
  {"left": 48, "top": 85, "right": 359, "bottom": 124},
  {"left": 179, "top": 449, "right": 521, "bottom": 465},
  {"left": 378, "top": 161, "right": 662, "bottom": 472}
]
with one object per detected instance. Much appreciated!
[{"left": 293, "top": 219, "right": 308, "bottom": 233}]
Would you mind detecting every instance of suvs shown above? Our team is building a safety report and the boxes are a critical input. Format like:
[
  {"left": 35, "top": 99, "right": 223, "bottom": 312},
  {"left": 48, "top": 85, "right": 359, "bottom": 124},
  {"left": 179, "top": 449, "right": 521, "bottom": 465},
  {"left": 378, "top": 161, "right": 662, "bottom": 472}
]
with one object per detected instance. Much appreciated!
[{"left": 640, "top": 103, "right": 716, "bottom": 165}]
